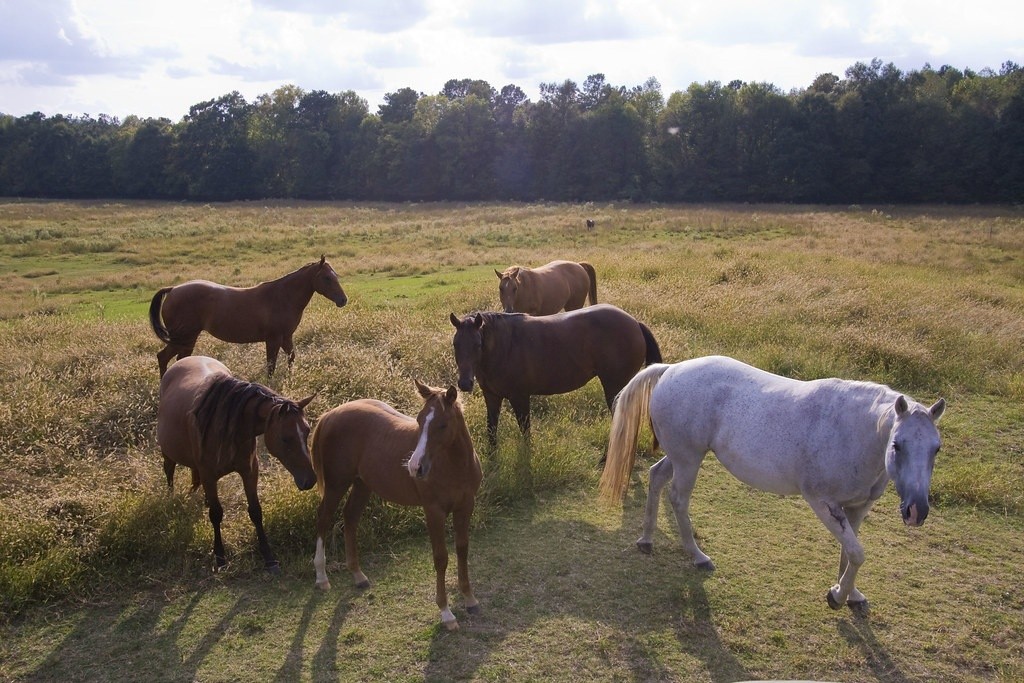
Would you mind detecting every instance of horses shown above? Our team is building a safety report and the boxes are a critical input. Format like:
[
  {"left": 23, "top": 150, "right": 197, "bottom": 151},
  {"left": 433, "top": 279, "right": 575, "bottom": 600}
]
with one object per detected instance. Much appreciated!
[
  {"left": 449, "top": 303, "right": 663, "bottom": 463},
  {"left": 494, "top": 259, "right": 598, "bottom": 317},
  {"left": 149, "top": 254, "right": 348, "bottom": 380},
  {"left": 157, "top": 356, "right": 317, "bottom": 575},
  {"left": 599, "top": 355, "right": 947, "bottom": 613},
  {"left": 311, "top": 379, "right": 482, "bottom": 631}
]
